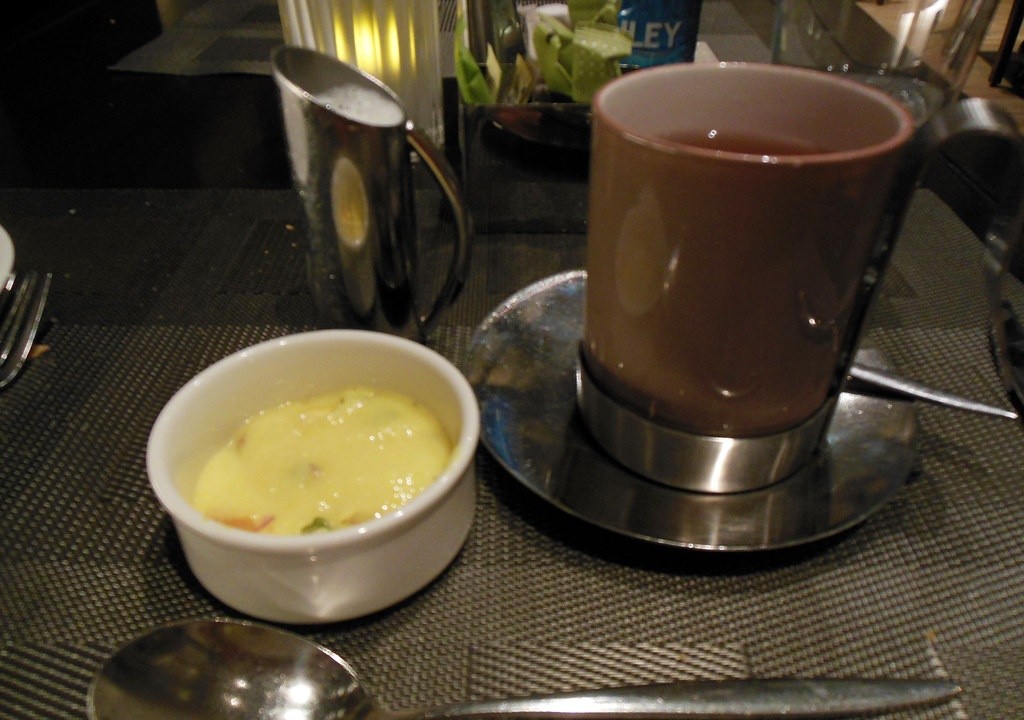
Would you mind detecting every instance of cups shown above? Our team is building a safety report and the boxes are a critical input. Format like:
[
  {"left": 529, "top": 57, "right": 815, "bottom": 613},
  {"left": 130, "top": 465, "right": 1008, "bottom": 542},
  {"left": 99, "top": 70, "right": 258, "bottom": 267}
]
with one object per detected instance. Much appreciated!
[
  {"left": 583, "top": 60, "right": 914, "bottom": 439},
  {"left": 771, "top": 0, "right": 1000, "bottom": 143},
  {"left": 270, "top": 42, "right": 470, "bottom": 343},
  {"left": 277, "top": 0, "right": 443, "bottom": 166}
]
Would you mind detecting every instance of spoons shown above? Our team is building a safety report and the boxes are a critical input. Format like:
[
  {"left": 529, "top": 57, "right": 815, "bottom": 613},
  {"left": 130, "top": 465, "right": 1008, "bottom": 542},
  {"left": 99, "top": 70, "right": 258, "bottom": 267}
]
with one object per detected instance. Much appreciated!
[{"left": 86, "top": 616, "right": 964, "bottom": 720}]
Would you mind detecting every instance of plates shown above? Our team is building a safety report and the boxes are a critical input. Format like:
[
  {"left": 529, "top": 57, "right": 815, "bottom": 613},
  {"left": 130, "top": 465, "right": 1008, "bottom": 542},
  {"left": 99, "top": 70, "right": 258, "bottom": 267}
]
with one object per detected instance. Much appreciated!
[
  {"left": 464, "top": 269, "right": 923, "bottom": 552},
  {"left": 0, "top": 224, "right": 16, "bottom": 296}
]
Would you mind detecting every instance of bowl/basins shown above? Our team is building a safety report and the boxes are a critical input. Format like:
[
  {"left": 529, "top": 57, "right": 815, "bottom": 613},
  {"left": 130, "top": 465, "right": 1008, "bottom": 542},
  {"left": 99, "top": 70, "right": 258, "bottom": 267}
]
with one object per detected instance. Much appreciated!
[
  {"left": 146, "top": 329, "right": 482, "bottom": 625},
  {"left": 330, "top": 156, "right": 377, "bottom": 316}
]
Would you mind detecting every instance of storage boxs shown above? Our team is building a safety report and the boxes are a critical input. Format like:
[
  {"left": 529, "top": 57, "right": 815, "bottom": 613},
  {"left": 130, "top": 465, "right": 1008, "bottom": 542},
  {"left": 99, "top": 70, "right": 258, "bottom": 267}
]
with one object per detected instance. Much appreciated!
[{"left": 455, "top": 64, "right": 640, "bottom": 238}]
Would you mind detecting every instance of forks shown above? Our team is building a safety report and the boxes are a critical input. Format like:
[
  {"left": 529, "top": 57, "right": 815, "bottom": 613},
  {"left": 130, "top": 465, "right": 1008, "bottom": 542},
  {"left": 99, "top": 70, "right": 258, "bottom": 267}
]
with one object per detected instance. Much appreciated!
[{"left": 0, "top": 271, "right": 53, "bottom": 388}]
